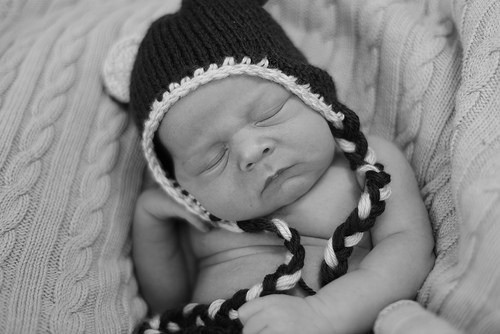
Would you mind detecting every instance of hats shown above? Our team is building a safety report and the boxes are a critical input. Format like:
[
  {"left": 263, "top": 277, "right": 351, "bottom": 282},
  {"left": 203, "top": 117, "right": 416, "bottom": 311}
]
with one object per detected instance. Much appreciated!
[{"left": 101, "top": 1, "right": 390, "bottom": 334}]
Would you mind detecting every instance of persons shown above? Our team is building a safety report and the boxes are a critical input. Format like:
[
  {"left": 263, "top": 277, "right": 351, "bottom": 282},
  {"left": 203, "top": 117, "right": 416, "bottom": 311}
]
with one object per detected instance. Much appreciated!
[{"left": 102, "top": 0, "right": 436, "bottom": 334}]
